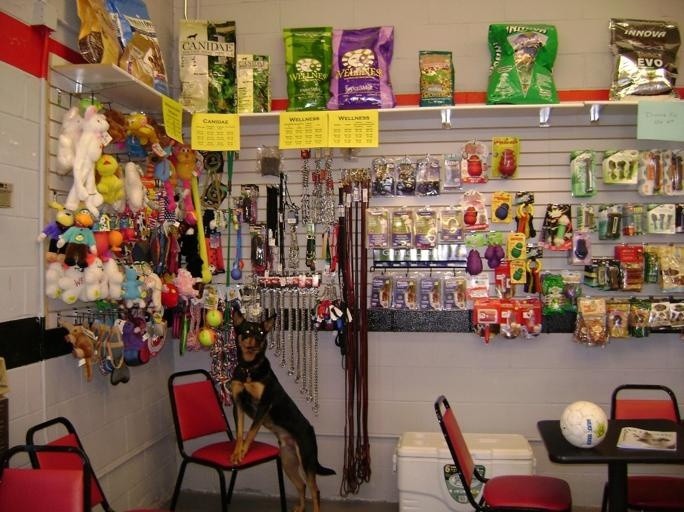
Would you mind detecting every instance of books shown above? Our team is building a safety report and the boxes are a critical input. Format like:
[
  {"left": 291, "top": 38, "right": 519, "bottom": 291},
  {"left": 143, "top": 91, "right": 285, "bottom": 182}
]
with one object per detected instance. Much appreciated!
[{"left": 615, "top": 426, "right": 677, "bottom": 451}]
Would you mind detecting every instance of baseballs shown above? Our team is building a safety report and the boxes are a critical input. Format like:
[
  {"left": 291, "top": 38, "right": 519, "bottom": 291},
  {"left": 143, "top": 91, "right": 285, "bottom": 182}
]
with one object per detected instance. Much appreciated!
[
  {"left": 198, "top": 329, "right": 215, "bottom": 346},
  {"left": 206, "top": 310, "right": 222, "bottom": 326}
]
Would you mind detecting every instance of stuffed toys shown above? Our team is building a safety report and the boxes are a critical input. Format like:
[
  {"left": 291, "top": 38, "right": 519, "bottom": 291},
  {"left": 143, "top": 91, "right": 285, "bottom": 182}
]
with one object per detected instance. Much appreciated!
[
  {"left": 38, "top": 105, "right": 214, "bottom": 386},
  {"left": 36, "top": 103, "right": 214, "bottom": 386}
]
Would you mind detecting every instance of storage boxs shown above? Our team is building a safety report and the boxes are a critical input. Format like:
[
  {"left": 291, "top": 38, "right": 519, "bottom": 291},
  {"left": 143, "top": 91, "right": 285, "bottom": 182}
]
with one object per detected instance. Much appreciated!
[{"left": 390, "top": 431, "right": 537, "bottom": 511}]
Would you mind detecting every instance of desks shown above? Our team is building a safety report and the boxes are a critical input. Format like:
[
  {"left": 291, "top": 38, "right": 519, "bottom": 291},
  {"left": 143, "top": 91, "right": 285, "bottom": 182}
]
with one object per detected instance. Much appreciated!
[{"left": 536, "top": 419, "right": 684, "bottom": 512}]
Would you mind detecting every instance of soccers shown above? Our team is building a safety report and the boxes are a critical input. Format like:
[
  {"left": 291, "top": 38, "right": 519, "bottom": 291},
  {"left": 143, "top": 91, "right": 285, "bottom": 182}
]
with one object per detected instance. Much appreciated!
[{"left": 560, "top": 400, "right": 608, "bottom": 448}]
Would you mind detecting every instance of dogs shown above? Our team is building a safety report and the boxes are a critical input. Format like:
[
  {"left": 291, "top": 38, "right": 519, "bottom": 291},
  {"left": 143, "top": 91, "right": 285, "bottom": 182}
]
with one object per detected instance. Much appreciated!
[{"left": 227, "top": 303, "right": 337, "bottom": 512}]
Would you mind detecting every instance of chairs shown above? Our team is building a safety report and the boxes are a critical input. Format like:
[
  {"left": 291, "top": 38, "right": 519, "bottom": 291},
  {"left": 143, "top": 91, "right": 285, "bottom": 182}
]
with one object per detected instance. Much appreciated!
[
  {"left": 600, "top": 384, "right": 683, "bottom": 512},
  {"left": 167, "top": 368, "right": 288, "bottom": 511},
  {"left": 434, "top": 395, "right": 573, "bottom": 512},
  {"left": 0, "top": 416, "right": 167, "bottom": 512}
]
